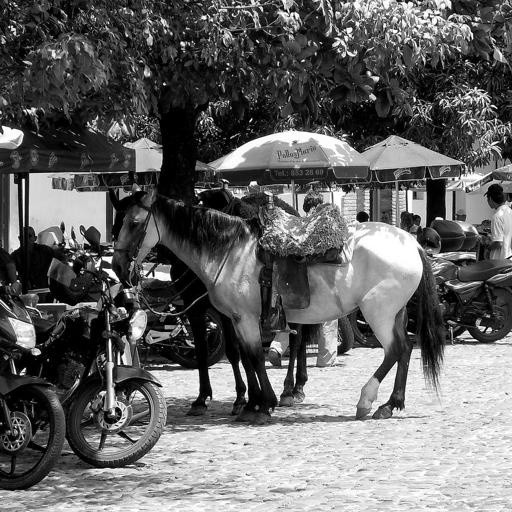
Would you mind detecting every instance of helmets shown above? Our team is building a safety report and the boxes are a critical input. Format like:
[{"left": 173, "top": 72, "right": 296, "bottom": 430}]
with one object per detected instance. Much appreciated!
[
  {"left": 47, "top": 257, "right": 95, "bottom": 306},
  {"left": 417, "top": 227, "right": 441, "bottom": 249}
]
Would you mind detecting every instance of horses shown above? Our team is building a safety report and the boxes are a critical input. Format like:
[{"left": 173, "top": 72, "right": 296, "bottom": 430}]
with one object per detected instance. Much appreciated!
[{"left": 108, "top": 181, "right": 448, "bottom": 426}]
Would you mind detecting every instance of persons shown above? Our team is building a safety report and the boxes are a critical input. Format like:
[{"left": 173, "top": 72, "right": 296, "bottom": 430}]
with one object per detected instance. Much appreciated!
[
  {"left": 268, "top": 195, "right": 347, "bottom": 365},
  {"left": 483, "top": 184, "right": 512, "bottom": 262},
  {"left": 400, "top": 210, "right": 421, "bottom": 232},
  {"left": 453, "top": 208, "right": 480, "bottom": 235},
  {"left": 0, "top": 240, "right": 16, "bottom": 286},
  {"left": 12, "top": 226, "right": 66, "bottom": 293},
  {"left": 356, "top": 212, "right": 369, "bottom": 222}
]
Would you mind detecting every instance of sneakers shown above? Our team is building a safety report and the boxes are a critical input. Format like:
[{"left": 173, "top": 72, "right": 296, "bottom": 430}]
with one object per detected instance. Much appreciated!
[{"left": 268, "top": 351, "right": 282, "bottom": 367}]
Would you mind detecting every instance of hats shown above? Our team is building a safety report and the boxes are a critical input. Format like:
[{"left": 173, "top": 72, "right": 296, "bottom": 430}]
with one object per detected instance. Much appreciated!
[
  {"left": 484, "top": 184, "right": 504, "bottom": 196},
  {"left": 79, "top": 225, "right": 101, "bottom": 248},
  {"left": 456, "top": 208, "right": 466, "bottom": 215}
]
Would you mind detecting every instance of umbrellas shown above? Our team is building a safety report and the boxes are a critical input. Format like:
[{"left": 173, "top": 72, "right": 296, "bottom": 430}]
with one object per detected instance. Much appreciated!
[
  {"left": 465, "top": 163, "right": 512, "bottom": 192},
  {"left": 0, "top": 113, "right": 136, "bottom": 291},
  {"left": 205, "top": 128, "right": 370, "bottom": 214},
  {"left": 48, "top": 137, "right": 218, "bottom": 241},
  {"left": 332, "top": 134, "right": 468, "bottom": 213}
]
